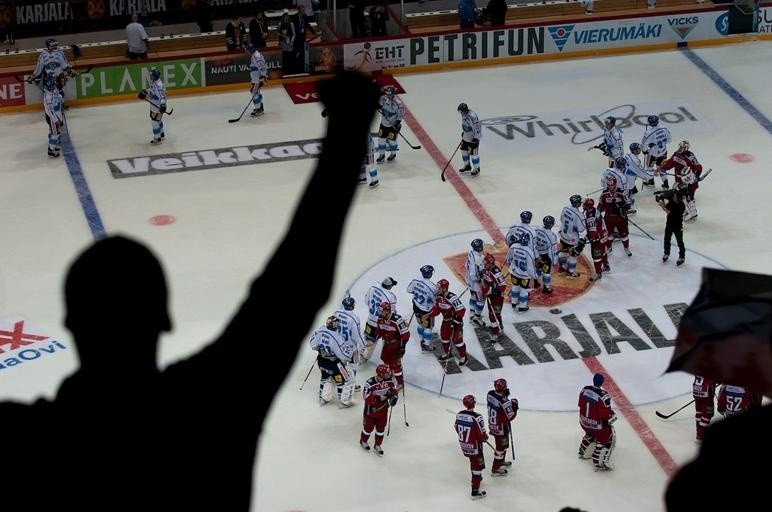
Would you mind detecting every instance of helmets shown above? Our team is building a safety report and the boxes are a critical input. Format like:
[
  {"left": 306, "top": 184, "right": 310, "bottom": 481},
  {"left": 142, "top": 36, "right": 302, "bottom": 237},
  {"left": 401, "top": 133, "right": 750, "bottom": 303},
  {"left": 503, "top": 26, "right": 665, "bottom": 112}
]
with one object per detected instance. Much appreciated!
[
  {"left": 607, "top": 175, "right": 616, "bottom": 186},
  {"left": 420, "top": 265, "right": 449, "bottom": 288},
  {"left": 570, "top": 195, "right": 594, "bottom": 209},
  {"left": 326, "top": 316, "right": 340, "bottom": 330},
  {"left": 520, "top": 211, "right": 555, "bottom": 245},
  {"left": 151, "top": 69, "right": 160, "bottom": 80},
  {"left": 593, "top": 373, "right": 604, "bottom": 384},
  {"left": 46, "top": 39, "right": 59, "bottom": 48},
  {"left": 494, "top": 379, "right": 506, "bottom": 393},
  {"left": 342, "top": 297, "right": 354, "bottom": 306},
  {"left": 458, "top": 103, "right": 468, "bottom": 113},
  {"left": 605, "top": 115, "right": 689, "bottom": 168},
  {"left": 463, "top": 395, "right": 474, "bottom": 408},
  {"left": 384, "top": 87, "right": 395, "bottom": 97},
  {"left": 377, "top": 302, "right": 391, "bottom": 314},
  {"left": 376, "top": 364, "right": 392, "bottom": 381},
  {"left": 245, "top": 44, "right": 255, "bottom": 54}
]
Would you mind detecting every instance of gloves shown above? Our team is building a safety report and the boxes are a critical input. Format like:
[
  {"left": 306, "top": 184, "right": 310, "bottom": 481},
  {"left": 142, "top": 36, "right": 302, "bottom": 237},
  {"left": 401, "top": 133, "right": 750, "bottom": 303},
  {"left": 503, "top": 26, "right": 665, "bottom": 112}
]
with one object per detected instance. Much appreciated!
[
  {"left": 394, "top": 123, "right": 401, "bottom": 133},
  {"left": 138, "top": 90, "right": 148, "bottom": 99},
  {"left": 470, "top": 138, "right": 479, "bottom": 148},
  {"left": 160, "top": 104, "right": 166, "bottom": 113}
]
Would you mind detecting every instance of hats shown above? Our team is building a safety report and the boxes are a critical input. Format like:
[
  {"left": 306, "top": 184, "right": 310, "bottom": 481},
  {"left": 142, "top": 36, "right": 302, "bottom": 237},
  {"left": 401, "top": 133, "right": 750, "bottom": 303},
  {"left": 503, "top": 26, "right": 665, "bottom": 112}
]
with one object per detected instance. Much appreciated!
[
  {"left": 382, "top": 277, "right": 397, "bottom": 289},
  {"left": 471, "top": 239, "right": 495, "bottom": 264}
]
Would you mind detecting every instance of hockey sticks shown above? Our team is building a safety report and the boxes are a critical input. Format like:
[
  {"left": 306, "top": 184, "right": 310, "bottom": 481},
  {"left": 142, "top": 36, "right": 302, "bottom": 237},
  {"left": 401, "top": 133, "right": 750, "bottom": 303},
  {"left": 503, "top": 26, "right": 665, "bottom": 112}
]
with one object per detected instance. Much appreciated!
[
  {"left": 588, "top": 146, "right": 617, "bottom": 160},
  {"left": 664, "top": 168, "right": 712, "bottom": 181},
  {"left": 654, "top": 399, "right": 694, "bottom": 418},
  {"left": 382, "top": 114, "right": 421, "bottom": 150},
  {"left": 441, "top": 140, "right": 461, "bottom": 181},
  {"left": 143, "top": 95, "right": 174, "bottom": 116},
  {"left": 400, "top": 379, "right": 410, "bottom": 426},
  {"left": 228, "top": 85, "right": 257, "bottom": 122},
  {"left": 12, "top": 70, "right": 77, "bottom": 84}
]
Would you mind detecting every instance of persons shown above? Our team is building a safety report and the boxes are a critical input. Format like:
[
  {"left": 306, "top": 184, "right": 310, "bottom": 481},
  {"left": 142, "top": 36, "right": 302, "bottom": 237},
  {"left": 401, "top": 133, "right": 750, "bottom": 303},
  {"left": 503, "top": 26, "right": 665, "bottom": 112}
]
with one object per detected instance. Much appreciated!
[
  {"left": 360, "top": 87, "right": 404, "bottom": 188},
  {"left": 348, "top": 1, "right": 367, "bottom": 37},
  {"left": 224, "top": 14, "right": 250, "bottom": 52},
  {"left": 245, "top": 43, "right": 268, "bottom": 118},
  {"left": 369, "top": 5, "right": 390, "bottom": 36},
  {"left": 482, "top": 1, "right": 509, "bottom": 25},
  {"left": 455, "top": 379, "right": 518, "bottom": 499},
  {"left": 309, "top": 276, "right": 410, "bottom": 455},
  {"left": 457, "top": 103, "right": 481, "bottom": 177},
  {"left": 577, "top": 374, "right": 616, "bottom": 470},
  {"left": 662, "top": 268, "right": 771, "bottom": 511},
  {"left": 3, "top": 66, "right": 380, "bottom": 512},
  {"left": 605, "top": 115, "right": 702, "bottom": 266},
  {"left": 275, "top": 13, "right": 297, "bottom": 76},
  {"left": 249, "top": 11, "right": 269, "bottom": 49},
  {"left": 27, "top": 39, "right": 77, "bottom": 159},
  {"left": 125, "top": 13, "right": 151, "bottom": 58},
  {"left": 290, "top": 5, "right": 318, "bottom": 73},
  {"left": 458, "top": 0, "right": 483, "bottom": 31},
  {"left": 506, "top": 156, "right": 633, "bottom": 312},
  {"left": 137, "top": 69, "right": 166, "bottom": 145},
  {"left": 407, "top": 239, "right": 507, "bottom": 366}
]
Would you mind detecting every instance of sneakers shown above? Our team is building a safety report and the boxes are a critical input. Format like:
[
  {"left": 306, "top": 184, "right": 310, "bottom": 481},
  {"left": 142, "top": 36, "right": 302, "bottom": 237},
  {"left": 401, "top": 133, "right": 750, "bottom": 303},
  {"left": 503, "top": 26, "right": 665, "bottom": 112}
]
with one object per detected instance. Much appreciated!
[
  {"left": 251, "top": 108, "right": 262, "bottom": 115},
  {"left": 682, "top": 210, "right": 696, "bottom": 220},
  {"left": 151, "top": 133, "right": 164, "bottom": 143},
  {"left": 559, "top": 268, "right": 580, "bottom": 277},
  {"left": 439, "top": 353, "right": 468, "bottom": 365},
  {"left": 459, "top": 165, "right": 479, "bottom": 175},
  {"left": 377, "top": 154, "right": 396, "bottom": 161},
  {"left": 48, "top": 148, "right": 60, "bottom": 156},
  {"left": 472, "top": 491, "right": 486, "bottom": 496},
  {"left": 470, "top": 316, "right": 486, "bottom": 326},
  {"left": 589, "top": 266, "right": 610, "bottom": 281},
  {"left": 625, "top": 250, "right": 632, "bottom": 256},
  {"left": 663, "top": 254, "right": 684, "bottom": 264},
  {"left": 512, "top": 303, "right": 529, "bottom": 311},
  {"left": 493, "top": 461, "right": 511, "bottom": 474},
  {"left": 360, "top": 440, "right": 383, "bottom": 454}
]
